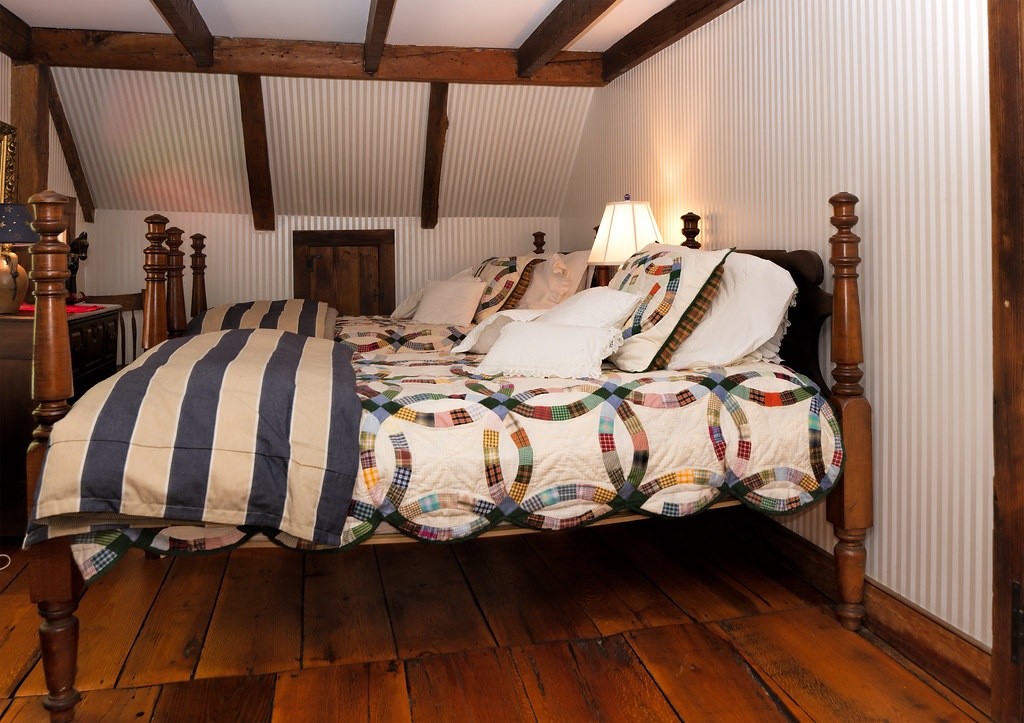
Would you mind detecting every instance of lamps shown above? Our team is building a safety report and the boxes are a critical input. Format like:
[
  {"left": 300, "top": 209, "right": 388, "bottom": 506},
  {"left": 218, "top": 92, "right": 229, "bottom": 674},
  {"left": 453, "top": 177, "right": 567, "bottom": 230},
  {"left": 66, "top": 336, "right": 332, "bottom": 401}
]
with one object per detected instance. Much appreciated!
[
  {"left": 0, "top": 204, "right": 40, "bottom": 314},
  {"left": 587, "top": 192, "right": 662, "bottom": 266}
]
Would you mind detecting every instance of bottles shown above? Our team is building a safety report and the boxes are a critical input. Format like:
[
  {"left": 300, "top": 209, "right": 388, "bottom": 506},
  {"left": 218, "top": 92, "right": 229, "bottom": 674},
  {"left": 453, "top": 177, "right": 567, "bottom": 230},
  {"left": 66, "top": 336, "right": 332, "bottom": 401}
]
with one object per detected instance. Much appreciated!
[{"left": 0, "top": 244, "right": 29, "bottom": 314}]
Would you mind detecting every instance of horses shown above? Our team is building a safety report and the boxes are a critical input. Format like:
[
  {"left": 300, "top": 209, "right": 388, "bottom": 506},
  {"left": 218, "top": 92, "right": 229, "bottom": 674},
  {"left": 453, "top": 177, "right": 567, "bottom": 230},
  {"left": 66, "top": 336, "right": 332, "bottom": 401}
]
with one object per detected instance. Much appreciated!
[{"left": 65, "top": 231, "right": 90, "bottom": 294}]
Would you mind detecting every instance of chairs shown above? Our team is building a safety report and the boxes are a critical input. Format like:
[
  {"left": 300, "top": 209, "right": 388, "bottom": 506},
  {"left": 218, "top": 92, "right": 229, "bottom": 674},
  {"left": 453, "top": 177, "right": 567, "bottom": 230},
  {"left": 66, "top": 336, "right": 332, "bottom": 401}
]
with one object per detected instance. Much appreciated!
[{"left": 80, "top": 289, "right": 146, "bottom": 372}]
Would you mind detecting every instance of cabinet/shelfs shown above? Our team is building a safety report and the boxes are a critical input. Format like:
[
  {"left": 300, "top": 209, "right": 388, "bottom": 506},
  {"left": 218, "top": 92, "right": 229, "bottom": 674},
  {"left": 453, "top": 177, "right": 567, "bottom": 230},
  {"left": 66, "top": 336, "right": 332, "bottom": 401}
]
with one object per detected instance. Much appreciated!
[{"left": 1, "top": 302, "right": 123, "bottom": 547}]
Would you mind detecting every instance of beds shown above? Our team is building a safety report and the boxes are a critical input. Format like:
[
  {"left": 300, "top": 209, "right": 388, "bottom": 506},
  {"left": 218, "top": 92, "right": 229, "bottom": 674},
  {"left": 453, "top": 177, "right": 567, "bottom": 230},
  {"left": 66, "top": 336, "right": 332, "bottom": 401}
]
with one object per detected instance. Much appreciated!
[
  {"left": 167, "top": 226, "right": 610, "bottom": 359},
  {"left": 18, "top": 191, "right": 875, "bottom": 722}
]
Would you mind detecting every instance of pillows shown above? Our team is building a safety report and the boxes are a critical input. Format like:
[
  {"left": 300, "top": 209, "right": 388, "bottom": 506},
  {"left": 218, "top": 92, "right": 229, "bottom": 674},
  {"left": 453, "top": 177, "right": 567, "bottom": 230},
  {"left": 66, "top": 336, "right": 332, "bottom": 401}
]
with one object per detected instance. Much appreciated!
[
  {"left": 518, "top": 254, "right": 570, "bottom": 308},
  {"left": 451, "top": 308, "right": 549, "bottom": 354},
  {"left": 471, "top": 255, "right": 545, "bottom": 326},
  {"left": 476, "top": 319, "right": 623, "bottom": 379},
  {"left": 546, "top": 287, "right": 642, "bottom": 330},
  {"left": 662, "top": 253, "right": 797, "bottom": 370},
  {"left": 449, "top": 267, "right": 482, "bottom": 281},
  {"left": 551, "top": 249, "right": 596, "bottom": 305},
  {"left": 411, "top": 281, "right": 486, "bottom": 327},
  {"left": 390, "top": 289, "right": 424, "bottom": 318},
  {"left": 602, "top": 241, "right": 737, "bottom": 374}
]
